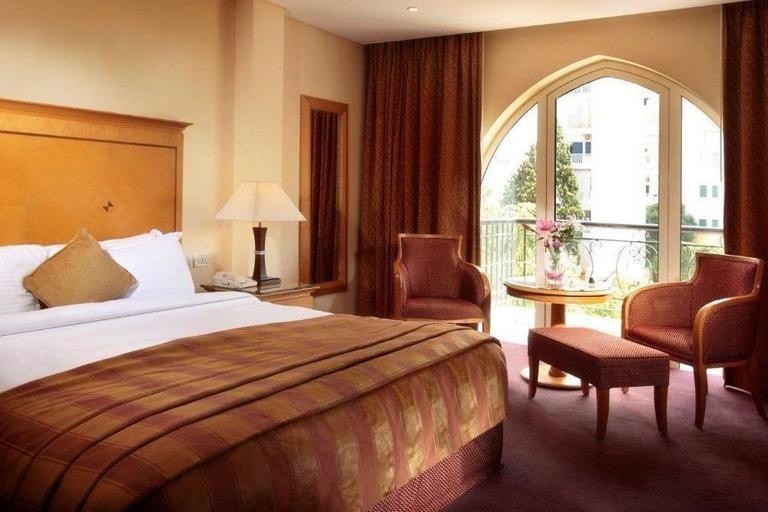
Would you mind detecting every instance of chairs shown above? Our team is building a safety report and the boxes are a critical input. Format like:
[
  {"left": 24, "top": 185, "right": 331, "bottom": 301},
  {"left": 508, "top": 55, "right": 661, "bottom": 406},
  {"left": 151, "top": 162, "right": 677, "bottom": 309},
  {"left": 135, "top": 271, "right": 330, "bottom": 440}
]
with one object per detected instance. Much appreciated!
[
  {"left": 393, "top": 234, "right": 490, "bottom": 333},
  {"left": 622, "top": 251, "right": 767, "bottom": 427}
]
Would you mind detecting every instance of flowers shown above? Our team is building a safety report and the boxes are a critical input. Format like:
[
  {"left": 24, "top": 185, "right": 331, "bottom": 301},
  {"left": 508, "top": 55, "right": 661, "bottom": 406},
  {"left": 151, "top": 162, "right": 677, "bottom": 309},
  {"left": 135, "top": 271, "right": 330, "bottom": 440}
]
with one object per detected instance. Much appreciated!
[{"left": 522, "top": 214, "right": 595, "bottom": 284}]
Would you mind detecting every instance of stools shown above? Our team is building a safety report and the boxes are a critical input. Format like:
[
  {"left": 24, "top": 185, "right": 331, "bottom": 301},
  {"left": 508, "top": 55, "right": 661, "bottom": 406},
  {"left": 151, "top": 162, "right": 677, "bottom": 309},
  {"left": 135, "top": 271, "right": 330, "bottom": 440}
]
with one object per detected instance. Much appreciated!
[{"left": 528, "top": 327, "right": 671, "bottom": 439}]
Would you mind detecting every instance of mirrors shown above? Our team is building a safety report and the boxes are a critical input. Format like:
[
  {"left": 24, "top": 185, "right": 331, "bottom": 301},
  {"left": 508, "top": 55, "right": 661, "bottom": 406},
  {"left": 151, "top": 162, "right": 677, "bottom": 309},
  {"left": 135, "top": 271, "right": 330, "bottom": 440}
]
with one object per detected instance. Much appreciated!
[{"left": 299, "top": 94, "right": 349, "bottom": 296}]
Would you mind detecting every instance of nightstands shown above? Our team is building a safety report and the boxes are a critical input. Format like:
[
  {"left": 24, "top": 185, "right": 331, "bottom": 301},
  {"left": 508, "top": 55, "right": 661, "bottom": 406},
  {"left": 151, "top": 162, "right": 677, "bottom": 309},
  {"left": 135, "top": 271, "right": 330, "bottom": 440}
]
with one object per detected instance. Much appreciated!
[{"left": 201, "top": 284, "right": 319, "bottom": 326}]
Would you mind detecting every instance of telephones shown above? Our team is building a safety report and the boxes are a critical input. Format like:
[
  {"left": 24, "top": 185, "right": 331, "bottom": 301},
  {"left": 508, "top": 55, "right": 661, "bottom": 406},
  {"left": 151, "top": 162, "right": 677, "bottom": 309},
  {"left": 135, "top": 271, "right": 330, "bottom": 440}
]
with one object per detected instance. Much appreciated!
[{"left": 212, "top": 270, "right": 258, "bottom": 290}]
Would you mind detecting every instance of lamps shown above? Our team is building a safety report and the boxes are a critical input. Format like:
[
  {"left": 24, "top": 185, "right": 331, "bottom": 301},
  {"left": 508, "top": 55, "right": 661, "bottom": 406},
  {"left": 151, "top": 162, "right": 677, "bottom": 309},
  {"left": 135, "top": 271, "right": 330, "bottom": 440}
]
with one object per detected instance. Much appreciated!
[{"left": 217, "top": 182, "right": 306, "bottom": 284}]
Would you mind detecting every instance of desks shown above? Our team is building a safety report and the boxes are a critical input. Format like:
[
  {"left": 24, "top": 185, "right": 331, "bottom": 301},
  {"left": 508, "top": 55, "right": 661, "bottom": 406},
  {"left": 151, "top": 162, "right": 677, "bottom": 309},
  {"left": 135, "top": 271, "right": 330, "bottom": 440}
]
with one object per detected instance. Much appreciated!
[{"left": 504, "top": 275, "right": 611, "bottom": 390}]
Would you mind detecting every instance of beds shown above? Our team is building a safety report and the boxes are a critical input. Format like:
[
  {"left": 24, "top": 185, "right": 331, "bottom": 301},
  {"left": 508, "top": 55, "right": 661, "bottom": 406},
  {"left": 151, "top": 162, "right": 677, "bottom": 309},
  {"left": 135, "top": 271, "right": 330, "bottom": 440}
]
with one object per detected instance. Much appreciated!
[{"left": 0, "top": 298, "right": 508, "bottom": 511}]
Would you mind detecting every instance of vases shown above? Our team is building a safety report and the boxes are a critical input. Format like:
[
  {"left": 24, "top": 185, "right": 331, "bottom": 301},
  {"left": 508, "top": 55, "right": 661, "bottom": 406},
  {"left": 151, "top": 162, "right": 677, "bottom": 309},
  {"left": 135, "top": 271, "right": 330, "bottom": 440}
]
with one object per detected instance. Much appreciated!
[{"left": 543, "top": 252, "right": 569, "bottom": 281}]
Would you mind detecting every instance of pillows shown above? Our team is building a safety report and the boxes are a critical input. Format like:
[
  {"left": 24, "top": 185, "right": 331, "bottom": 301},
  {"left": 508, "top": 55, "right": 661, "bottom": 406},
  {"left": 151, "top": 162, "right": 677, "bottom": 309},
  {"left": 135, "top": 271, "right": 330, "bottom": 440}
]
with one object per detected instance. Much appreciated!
[
  {"left": 99, "top": 232, "right": 195, "bottom": 309},
  {"left": 0, "top": 244, "right": 44, "bottom": 315},
  {"left": 49, "top": 230, "right": 163, "bottom": 259},
  {"left": 21, "top": 227, "right": 139, "bottom": 307}
]
